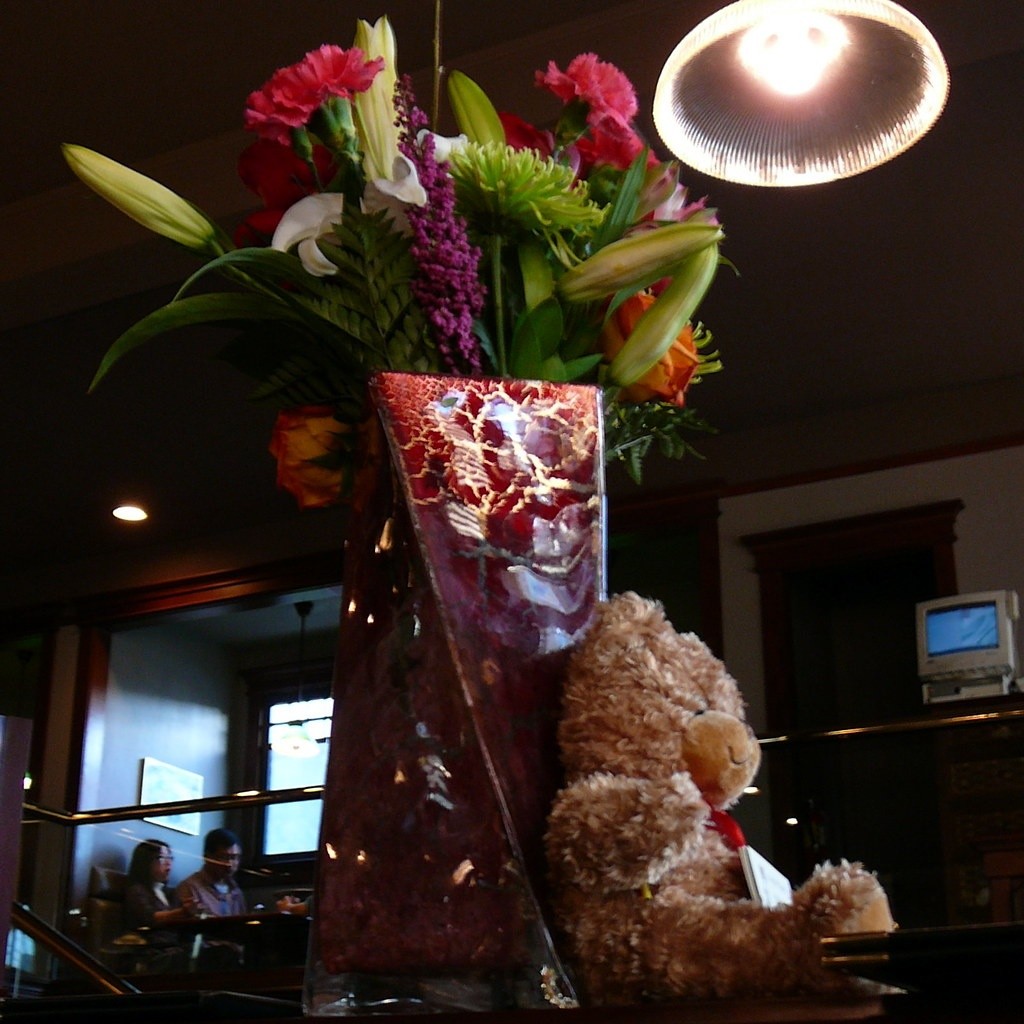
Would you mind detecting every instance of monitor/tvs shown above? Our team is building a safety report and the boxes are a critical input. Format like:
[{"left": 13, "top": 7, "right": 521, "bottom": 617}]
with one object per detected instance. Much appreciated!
[{"left": 916, "top": 589, "right": 1021, "bottom": 704}]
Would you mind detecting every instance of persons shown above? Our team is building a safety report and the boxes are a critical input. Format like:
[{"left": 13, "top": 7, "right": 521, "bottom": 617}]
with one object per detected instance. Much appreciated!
[
  {"left": 172, "top": 828, "right": 305, "bottom": 1002},
  {"left": 126, "top": 838, "right": 261, "bottom": 997},
  {"left": 277, "top": 884, "right": 315, "bottom": 921}
]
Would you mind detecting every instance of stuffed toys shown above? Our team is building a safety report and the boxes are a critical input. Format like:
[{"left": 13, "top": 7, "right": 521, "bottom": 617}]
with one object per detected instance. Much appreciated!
[{"left": 537, "top": 589, "right": 907, "bottom": 1009}]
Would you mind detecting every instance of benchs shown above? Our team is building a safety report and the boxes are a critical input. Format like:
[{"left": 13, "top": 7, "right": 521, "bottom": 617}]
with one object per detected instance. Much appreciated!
[{"left": 80, "top": 866, "right": 182, "bottom": 966}]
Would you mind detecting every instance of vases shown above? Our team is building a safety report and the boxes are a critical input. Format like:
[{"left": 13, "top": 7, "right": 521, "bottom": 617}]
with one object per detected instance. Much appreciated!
[{"left": 297, "top": 501, "right": 589, "bottom": 1023}]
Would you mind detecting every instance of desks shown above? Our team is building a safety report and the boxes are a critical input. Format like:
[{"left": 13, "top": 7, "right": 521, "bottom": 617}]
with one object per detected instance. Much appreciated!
[
  {"left": 187, "top": 907, "right": 313, "bottom": 949},
  {"left": 63, "top": 15, "right": 744, "bottom": 973}
]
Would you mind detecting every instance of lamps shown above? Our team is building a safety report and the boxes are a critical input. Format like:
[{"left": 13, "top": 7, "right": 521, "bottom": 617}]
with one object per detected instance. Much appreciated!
[{"left": 653, "top": 0, "right": 950, "bottom": 189}]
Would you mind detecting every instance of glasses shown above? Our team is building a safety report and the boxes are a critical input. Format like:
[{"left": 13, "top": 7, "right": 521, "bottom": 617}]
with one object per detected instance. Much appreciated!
[{"left": 152, "top": 854, "right": 174, "bottom": 865}]
